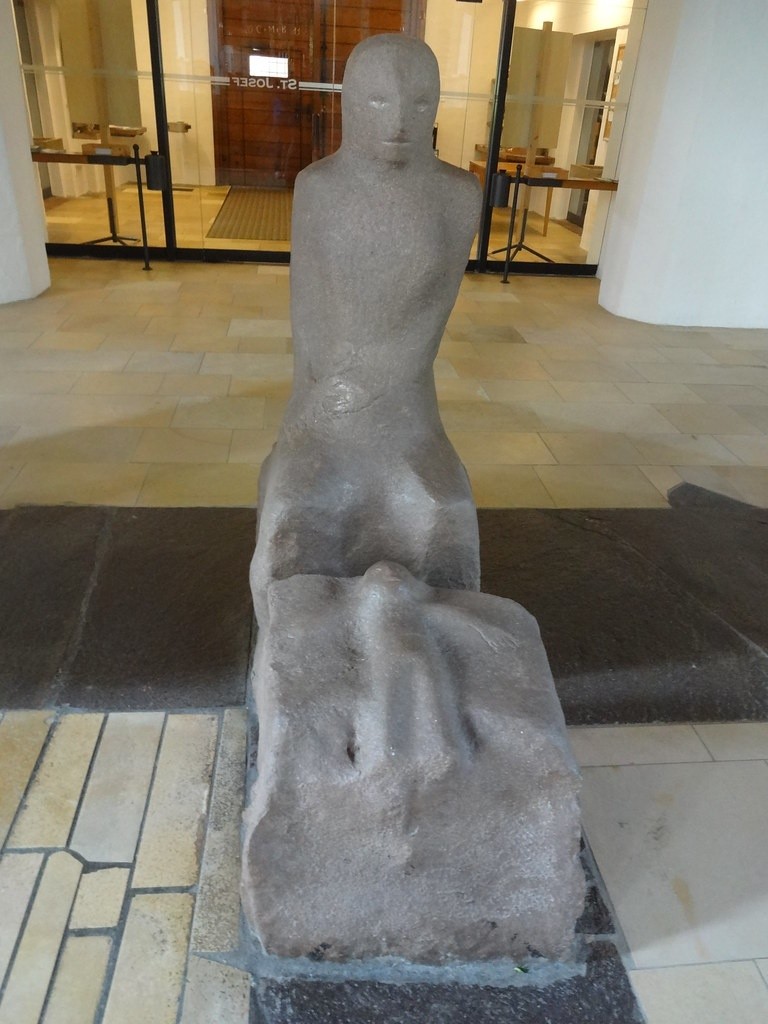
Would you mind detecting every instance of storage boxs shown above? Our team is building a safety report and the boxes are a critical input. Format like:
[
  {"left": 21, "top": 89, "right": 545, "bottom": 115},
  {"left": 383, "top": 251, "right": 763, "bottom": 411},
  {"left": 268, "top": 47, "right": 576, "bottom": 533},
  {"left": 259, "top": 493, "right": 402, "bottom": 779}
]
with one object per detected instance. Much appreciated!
[
  {"left": 524, "top": 163, "right": 569, "bottom": 180},
  {"left": 570, "top": 163, "right": 603, "bottom": 178},
  {"left": 82, "top": 143, "right": 131, "bottom": 157},
  {"left": 33, "top": 137, "right": 63, "bottom": 150}
]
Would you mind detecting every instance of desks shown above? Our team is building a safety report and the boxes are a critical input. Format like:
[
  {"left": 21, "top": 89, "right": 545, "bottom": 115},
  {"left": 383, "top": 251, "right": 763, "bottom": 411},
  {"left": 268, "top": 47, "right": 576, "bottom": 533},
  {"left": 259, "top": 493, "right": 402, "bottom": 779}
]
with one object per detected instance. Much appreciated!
[
  {"left": 468, "top": 160, "right": 618, "bottom": 263},
  {"left": 469, "top": 160, "right": 553, "bottom": 237},
  {"left": 474, "top": 144, "right": 555, "bottom": 165}
]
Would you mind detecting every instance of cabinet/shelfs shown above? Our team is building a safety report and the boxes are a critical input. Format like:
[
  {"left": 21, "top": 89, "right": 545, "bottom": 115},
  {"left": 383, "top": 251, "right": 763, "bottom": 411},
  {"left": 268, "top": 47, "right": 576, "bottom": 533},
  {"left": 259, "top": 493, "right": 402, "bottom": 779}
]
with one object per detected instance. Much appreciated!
[{"left": 485, "top": 27, "right": 574, "bottom": 149}]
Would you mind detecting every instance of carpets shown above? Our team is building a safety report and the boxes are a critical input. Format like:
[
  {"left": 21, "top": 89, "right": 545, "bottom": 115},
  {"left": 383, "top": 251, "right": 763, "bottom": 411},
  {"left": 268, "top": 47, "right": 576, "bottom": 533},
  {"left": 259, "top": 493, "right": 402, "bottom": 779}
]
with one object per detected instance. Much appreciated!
[{"left": 205, "top": 186, "right": 293, "bottom": 241}]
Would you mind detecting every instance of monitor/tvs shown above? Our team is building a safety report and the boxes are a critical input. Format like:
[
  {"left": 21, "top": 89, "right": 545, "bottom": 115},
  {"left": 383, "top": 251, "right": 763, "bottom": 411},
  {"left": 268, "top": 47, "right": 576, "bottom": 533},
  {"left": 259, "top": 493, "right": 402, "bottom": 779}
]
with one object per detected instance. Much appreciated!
[{"left": 249, "top": 55, "right": 288, "bottom": 79}]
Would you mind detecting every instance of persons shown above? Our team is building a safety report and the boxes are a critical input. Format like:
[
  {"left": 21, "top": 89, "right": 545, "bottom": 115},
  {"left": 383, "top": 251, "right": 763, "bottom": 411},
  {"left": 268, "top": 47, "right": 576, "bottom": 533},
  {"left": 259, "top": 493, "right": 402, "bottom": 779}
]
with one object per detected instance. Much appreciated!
[{"left": 247, "top": 33, "right": 484, "bottom": 586}]
